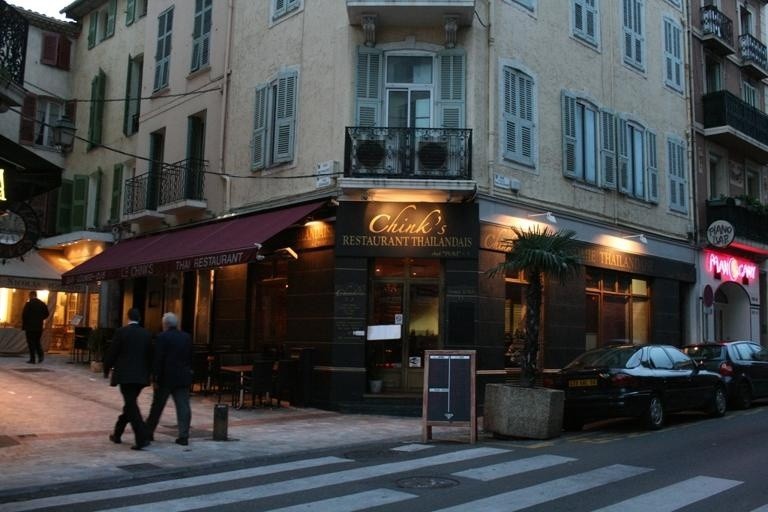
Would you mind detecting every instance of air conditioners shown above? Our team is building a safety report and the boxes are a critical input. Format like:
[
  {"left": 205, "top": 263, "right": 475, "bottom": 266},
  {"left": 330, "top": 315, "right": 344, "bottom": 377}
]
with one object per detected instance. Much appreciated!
[{"left": 349, "top": 132, "right": 463, "bottom": 180}]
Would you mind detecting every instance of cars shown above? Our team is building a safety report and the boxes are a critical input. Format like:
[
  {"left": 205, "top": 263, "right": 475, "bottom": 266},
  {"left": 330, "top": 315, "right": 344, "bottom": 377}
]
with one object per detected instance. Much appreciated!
[
  {"left": 544, "top": 342, "right": 728, "bottom": 431},
  {"left": 679, "top": 339, "right": 767, "bottom": 409}
]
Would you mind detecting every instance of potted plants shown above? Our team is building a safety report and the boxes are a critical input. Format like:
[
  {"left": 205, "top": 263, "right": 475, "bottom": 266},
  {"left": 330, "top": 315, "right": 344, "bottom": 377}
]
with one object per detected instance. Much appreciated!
[{"left": 485, "top": 227, "right": 580, "bottom": 439}]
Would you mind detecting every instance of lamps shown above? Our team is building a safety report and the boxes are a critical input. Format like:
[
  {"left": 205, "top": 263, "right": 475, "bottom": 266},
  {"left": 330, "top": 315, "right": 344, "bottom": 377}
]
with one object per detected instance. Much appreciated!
[
  {"left": 527, "top": 210, "right": 558, "bottom": 224},
  {"left": 621, "top": 234, "right": 648, "bottom": 244},
  {"left": 49, "top": 114, "right": 77, "bottom": 157}
]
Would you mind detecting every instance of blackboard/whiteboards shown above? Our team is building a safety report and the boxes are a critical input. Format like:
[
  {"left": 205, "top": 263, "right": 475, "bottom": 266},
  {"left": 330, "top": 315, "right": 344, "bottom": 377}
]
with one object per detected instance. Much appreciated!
[{"left": 422, "top": 350, "right": 476, "bottom": 426}]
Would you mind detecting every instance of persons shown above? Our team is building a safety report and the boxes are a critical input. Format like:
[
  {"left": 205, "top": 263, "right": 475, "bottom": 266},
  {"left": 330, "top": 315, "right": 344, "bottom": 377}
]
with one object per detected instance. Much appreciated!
[
  {"left": 103, "top": 308, "right": 153, "bottom": 450},
  {"left": 145, "top": 311, "right": 196, "bottom": 447},
  {"left": 21, "top": 290, "right": 50, "bottom": 364}
]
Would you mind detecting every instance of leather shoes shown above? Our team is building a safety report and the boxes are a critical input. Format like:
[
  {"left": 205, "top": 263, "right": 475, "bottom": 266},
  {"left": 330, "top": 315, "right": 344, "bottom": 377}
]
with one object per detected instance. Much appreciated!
[
  {"left": 110, "top": 434, "right": 122, "bottom": 444},
  {"left": 129, "top": 432, "right": 154, "bottom": 449},
  {"left": 175, "top": 437, "right": 189, "bottom": 445},
  {"left": 26, "top": 357, "right": 45, "bottom": 364}
]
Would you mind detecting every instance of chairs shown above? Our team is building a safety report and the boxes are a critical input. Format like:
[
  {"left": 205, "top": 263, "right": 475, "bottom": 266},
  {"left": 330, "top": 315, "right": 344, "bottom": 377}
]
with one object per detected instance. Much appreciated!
[
  {"left": 194, "top": 344, "right": 294, "bottom": 412},
  {"left": 72, "top": 327, "right": 93, "bottom": 364}
]
